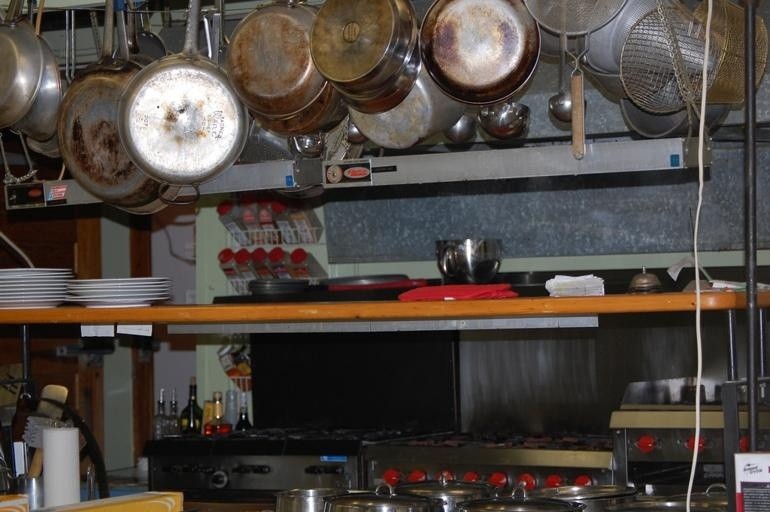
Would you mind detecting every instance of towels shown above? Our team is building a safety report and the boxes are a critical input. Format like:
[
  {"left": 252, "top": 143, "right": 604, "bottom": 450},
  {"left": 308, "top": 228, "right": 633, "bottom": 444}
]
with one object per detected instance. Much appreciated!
[{"left": 399, "top": 284, "right": 519, "bottom": 299}]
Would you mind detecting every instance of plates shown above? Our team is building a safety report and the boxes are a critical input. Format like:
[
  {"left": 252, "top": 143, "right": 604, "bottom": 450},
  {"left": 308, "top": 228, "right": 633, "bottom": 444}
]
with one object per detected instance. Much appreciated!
[
  {"left": 320, "top": 273, "right": 408, "bottom": 289},
  {"left": 0, "top": 267, "right": 74, "bottom": 310},
  {"left": 248, "top": 277, "right": 311, "bottom": 296},
  {"left": 65, "top": 275, "right": 172, "bottom": 308}
]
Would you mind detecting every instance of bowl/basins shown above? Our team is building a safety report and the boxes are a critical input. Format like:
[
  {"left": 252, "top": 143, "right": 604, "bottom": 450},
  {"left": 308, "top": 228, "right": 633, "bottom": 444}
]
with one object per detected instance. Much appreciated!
[{"left": 435, "top": 236, "right": 502, "bottom": 284}]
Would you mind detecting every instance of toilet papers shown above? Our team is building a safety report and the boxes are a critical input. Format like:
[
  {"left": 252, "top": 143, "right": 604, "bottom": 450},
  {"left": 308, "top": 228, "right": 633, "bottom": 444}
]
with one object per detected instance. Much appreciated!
[{"left": 41, "top": 426, "right": 80, "bottom": 508}]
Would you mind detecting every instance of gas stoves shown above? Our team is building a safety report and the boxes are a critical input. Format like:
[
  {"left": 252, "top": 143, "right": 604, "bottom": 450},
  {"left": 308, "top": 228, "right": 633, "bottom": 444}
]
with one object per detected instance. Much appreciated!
[
  {"left": 361, "top": 421, "right": 613, "bottom": 489},
  {"left": 147, "top": 426, "right": 454, "bottom": 502},
  {"left": 607, "top": 378, "right": 770, "bottom": 465}
]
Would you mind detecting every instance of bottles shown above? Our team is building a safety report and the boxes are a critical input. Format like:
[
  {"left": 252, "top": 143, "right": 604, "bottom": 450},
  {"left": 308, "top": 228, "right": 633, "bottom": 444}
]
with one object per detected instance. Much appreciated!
[
  {"left": 0, "top": 464, "right": 44, "bottom": 512},
  {"left": 213, "top": 197, "right": 325, "bottom": 246},
  {"left": 152, "top": 375, "right": 252, "bottom": 439},
  {"left": 215, "top": 248, "right": 328, "bottom": 296}
]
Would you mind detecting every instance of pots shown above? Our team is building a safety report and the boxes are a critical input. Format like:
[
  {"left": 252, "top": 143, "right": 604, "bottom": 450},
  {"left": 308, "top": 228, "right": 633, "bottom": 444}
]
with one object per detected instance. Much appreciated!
[
  {"left": 274, "top": 474, "right": 727, "bottom": 511},
  {"left": 1, "top": 2, "right": 544, "bottom": 222}
]
